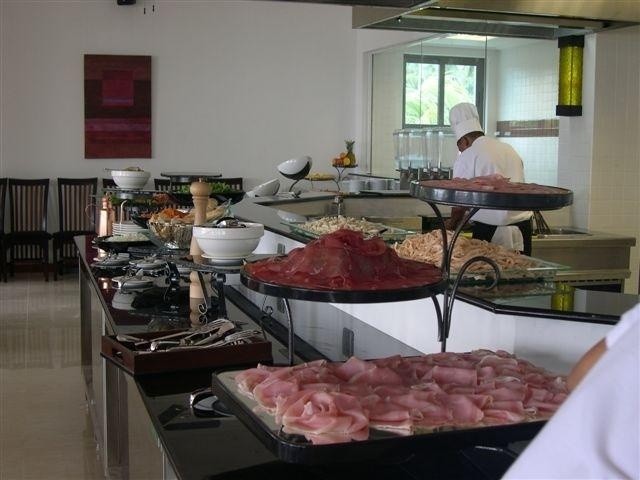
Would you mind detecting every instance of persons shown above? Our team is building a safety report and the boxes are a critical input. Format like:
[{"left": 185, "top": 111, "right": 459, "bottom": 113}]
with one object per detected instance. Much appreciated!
[
  {"left": 442, "top": 119, "right": 533, "bottom": 256},
  {"left": 499, "top": 301, "right": 640, "bottom": 480}
]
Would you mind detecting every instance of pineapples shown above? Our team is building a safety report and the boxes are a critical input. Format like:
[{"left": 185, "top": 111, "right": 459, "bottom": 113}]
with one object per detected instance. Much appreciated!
[{"left": 346, "top": 140, "right": 356, "bottom": 165}]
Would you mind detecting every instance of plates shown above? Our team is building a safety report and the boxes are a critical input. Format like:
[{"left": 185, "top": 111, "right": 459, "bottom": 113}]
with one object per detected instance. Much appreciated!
[
  {"left": 112, "top": 275, "right": 156, "bottom": 288},
  {"left": 340, "top": 177, "right": 400, "bottom": 194},
  {"left": 128, "top": 260, "right": 167, "bottom": 269},
  {"left": 200, "top": 253, "right": 246, "bottom": 266},
  {"left": 111, "top": 222, "right": 143, "bottom": 236}
]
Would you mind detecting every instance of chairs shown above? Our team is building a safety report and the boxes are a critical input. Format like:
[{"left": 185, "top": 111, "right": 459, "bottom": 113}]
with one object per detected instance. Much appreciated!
[{"left": 0, "top": 173, "right": 243, "bottom": 283}]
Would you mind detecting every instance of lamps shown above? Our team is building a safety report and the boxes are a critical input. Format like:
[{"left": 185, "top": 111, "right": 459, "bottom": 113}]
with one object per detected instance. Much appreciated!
[{"left": 556, "top": 37, "right": 586, "bottom": 117}]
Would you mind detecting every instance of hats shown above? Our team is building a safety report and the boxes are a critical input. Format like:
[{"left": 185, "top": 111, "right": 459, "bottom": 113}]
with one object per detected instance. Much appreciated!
[{"left": 447, "top": 101, "right": 484, "bottom": 144}]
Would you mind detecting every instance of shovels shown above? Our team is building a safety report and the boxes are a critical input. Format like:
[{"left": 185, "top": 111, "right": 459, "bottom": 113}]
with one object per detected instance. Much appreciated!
[{"left": 135, "top": 318, "right": 263, "bottom": 353}]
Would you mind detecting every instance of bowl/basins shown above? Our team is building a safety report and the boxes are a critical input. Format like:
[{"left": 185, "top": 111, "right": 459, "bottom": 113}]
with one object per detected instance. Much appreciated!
[
  {"left": 110, "top": 171, "right": 151, "bottom": 189},
  {"left": 253, "top": 177, "right": 279, "bottom": 196},
  {"left": 277, "top": 155, "right": 313, "bottom": 175},
  {"left": 112, "top": 290, "right": 138, "bottom": 311},
  {"left": 192, "top": 221, "right": 265, "bottom": 256}
]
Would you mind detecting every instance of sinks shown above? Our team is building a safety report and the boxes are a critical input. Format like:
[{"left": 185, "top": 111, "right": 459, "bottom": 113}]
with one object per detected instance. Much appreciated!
[{"left": 533, "top": 224, "right": 587, "bottom": 238}]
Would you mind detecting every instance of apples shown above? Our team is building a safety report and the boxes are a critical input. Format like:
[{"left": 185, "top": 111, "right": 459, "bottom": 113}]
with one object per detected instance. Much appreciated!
[{"left": 332, "top": 152, "right": 350, "bottom": 165}]
